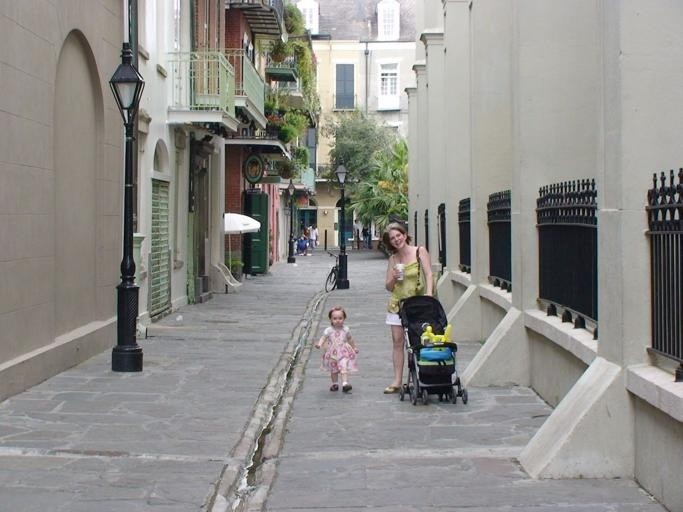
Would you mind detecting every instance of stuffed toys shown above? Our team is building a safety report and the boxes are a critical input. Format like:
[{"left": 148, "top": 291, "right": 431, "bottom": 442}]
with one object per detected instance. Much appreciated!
[{"left": 421, "top": 324, "right": 452, "bottom": 351}]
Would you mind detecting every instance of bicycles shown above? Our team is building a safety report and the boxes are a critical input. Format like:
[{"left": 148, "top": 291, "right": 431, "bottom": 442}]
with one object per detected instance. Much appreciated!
[
  {"left": 297, "top": 240, "right": 308, "bottom": 255},
  {"left": 325, "top": 251, "right": 339, "bottom": 292}
]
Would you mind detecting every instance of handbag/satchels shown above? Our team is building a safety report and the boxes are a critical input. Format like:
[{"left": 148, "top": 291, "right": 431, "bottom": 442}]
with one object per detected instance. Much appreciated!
[{"left": 414, "top": 245, "right": 439, "bottom": 302}]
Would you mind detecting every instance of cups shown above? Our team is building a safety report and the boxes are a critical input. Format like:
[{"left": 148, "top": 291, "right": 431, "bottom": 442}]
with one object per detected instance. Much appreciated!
[{"left": 394, "top": 263, "right": 404, "bottom": 281}]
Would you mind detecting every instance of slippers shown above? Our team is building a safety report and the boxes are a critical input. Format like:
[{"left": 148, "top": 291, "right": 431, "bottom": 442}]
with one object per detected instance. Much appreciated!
[{"left": 383, "top": 384, "right": 401, "bottom": 394}]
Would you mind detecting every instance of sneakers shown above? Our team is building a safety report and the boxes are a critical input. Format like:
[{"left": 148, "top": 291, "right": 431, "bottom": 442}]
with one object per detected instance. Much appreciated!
[
  {"left": 330, "top": 383, "right": 338, "bottom": 391},
  {"left": 342, "top": 383, "right": 352, "bottom": 392}
]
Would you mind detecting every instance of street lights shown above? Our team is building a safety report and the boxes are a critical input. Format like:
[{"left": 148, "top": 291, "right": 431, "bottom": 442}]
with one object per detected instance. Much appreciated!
[
  {"left": 287, "top": 179, "right": 295, "bottom": 263},
  {"left": 335, "top": 156, "right": 349, "bottom": 289},
  {"left": 108, "top": 41, "right": 146, "bottom": 372}
]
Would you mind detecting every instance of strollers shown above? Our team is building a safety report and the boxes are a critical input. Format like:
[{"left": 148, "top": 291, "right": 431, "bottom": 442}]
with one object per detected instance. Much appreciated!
[{"left": 399, "top": 295, "right": 469, "bottom": 406}]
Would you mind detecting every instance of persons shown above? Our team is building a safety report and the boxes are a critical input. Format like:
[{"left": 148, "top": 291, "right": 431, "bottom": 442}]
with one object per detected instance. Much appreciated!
[
  {"left": 383, "top": 222, "right": 435, "bottom": 394},
  {"left": 298, "top": 223, "right": 319, "bottom": 256},
  {"left": 362, "top": 224, "right": 370, "bottom": 248},
  {"left": 314, "top": 306, "right": 359, "bottom": 392}
]
